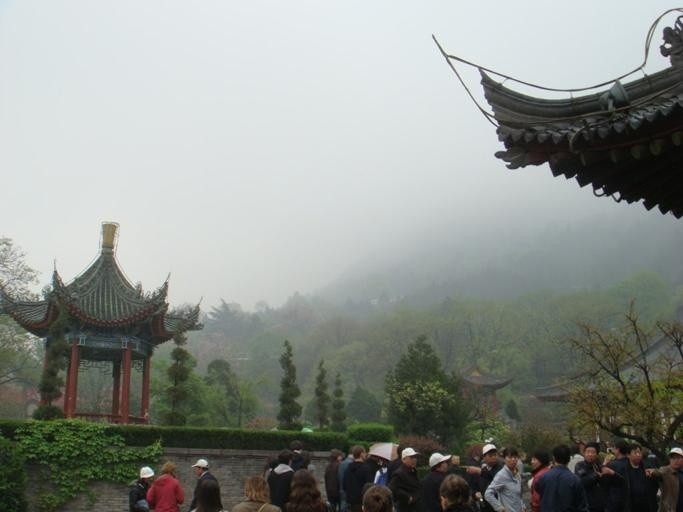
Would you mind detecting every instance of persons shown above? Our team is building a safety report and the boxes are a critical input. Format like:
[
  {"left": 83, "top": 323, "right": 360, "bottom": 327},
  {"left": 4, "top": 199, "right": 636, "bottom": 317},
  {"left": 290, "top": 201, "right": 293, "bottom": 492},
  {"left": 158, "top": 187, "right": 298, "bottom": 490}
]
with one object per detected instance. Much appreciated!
[
  {"left": 127, "top": 466, "right": 154, "bottom": 512},
  {"left": 191, "top": 478, "right": 228, "bottom": 512},
  {"left": 144, "top": 461, "right": 185, "bottom": 512},
  {"left": 187, "top": 459, "right": 225, "bottom": 512}
]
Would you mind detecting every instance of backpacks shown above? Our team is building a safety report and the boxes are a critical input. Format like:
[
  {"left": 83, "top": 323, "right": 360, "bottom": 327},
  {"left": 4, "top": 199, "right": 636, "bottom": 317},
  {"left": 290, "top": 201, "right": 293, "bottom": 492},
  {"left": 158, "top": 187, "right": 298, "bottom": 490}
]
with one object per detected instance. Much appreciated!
[{"left": 376, "top": 468, "right": 390, "bottom": 487}]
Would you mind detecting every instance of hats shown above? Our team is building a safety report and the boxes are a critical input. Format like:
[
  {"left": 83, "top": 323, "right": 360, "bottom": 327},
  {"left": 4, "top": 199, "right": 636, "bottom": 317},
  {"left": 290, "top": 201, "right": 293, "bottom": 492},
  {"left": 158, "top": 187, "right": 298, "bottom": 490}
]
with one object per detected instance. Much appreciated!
[
  {"left": 667, "top": 446, "right": 683, "bottom": 457},
  {"left": 190, "top": 458, "right": 209, "bottom": 469},
  {"left": 482, "top": 444, "right": 498, "bottom": 455},
  {"left": 138, "top": 466, "right": 155, "bottom": 480},
  {"left": 401, "top": 447, "right": 422, "bottom": 459},
  {"left": 428, "top": 452, "right": 453, "bottom": 468}
]
